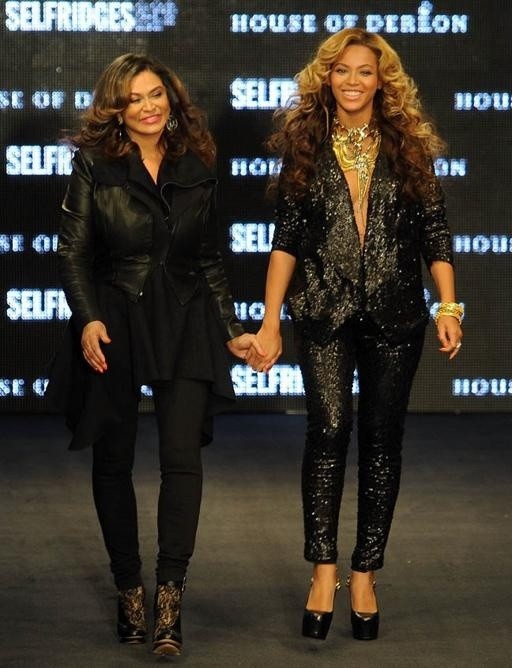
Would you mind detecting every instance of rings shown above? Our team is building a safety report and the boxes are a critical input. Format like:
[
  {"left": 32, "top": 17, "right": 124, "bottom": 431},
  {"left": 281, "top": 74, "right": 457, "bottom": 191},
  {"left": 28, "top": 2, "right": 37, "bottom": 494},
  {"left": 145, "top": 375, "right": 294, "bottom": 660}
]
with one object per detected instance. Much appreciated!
[{"left": 456, "top": 341, "right": 463, "bottom": 350}]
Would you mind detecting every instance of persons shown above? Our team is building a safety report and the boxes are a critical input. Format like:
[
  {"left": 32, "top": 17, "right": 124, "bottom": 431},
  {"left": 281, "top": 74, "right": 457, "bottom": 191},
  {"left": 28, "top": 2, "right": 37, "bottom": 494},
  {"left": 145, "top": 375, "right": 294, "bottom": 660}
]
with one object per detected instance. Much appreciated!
[
  {"left": 57, "top": 52, "right": 266, "bottom": 659},
  {"left": 245, "top": 28, "right": 466, "bottom": 643}
]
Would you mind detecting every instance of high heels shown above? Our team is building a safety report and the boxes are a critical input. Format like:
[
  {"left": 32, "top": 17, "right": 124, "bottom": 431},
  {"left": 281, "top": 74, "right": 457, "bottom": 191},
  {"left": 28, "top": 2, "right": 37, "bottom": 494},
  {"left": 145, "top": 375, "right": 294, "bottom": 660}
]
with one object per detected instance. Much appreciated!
[
  {"left": 346, "top": 574, "right": 380, "bottom": 640},
  {"left": 303, "top": 576, "right": 340, "bottom": 641}
]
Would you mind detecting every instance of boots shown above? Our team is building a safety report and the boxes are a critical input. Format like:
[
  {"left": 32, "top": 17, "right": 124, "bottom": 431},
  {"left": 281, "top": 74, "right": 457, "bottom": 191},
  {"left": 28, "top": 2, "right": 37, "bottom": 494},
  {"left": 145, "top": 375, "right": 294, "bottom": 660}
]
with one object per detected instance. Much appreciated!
[
  {"left": 117, "top": 585, "right": 148, "bottom": 646},
  {"left": 151, "top": 577, "right": 186, "bottom": 656}
]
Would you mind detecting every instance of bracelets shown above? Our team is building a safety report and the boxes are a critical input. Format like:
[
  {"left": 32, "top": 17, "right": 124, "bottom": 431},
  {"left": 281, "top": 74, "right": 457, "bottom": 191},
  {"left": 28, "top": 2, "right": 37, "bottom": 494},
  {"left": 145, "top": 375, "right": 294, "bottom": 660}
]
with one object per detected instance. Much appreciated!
[{"left": 433, "top": 299, "right": 465, "bottom": 326}]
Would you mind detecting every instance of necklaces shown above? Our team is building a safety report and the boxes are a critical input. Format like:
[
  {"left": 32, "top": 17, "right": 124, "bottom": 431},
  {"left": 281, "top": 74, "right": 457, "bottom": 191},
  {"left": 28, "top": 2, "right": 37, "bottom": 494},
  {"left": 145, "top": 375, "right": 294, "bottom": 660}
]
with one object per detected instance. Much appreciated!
[{"left": 330, "top": 117, "right": 381, "bottom": 204}]
